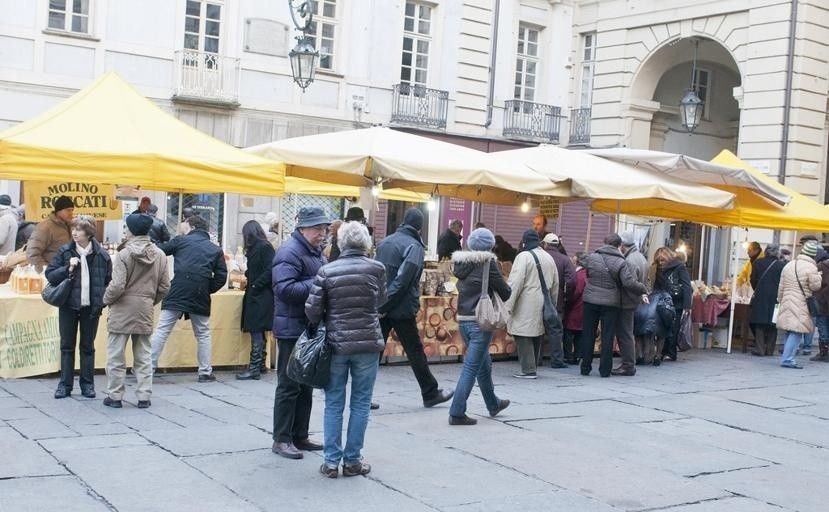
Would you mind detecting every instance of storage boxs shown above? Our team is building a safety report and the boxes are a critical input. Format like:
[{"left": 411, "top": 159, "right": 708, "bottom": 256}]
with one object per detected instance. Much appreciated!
[{"left": 692, "top": 319, "right": 727, "bottom": 349}]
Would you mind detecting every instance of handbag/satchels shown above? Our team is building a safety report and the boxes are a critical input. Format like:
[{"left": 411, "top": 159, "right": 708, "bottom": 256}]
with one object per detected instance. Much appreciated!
[
  {"left": 749, "top": 292, "right": 758, "bottom": 309},
  {"left": 41, "top": 273, "right": 76, "bottom": 307},
  {"left": 806, "top": 295, "right": 820, "bottom": 317},
  {"left": 656, "top": 298, "right": 676, "bottom": 329},
  {"left": 285, "top": 320, "right": 332, "bottom": 390},
  {"left": 676, "top": 310, "right": 695, "bottom": 352},
  {"left": 475, "top": 258, "right": 510, "bottom": 331},
  {"left": 543, "top": 292, "right": 564, "bottom": 339}
]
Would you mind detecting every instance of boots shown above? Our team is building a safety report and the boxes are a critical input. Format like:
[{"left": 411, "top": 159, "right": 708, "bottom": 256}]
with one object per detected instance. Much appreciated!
[
  {"left": 236, "top": 344, "right": 263, "bottom": 380},
  {"left": 247, "top": 343, "right": 266, "bottom": 373}
]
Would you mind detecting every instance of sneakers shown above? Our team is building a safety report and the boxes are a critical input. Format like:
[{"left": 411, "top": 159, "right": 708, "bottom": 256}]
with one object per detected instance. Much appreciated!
[
  {"left": 343, "top": 461, "right": 371, "bottom": 477},
  {"left": 320, "top": 463, "right": 338, "bottom": 478},
  {"left": 515, "top": 372, "right": 537, "bottom": 379},
  {"left": 803, "top": 349, "right": 811, "bottom": 354}
]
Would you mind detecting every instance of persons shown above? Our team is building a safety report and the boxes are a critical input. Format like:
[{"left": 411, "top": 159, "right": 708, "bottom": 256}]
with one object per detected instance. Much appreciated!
[
  {"left": 270, "top": 205, "right": 328, "bottom": 459},
  {"left": 736, "top": 234, "right": 828, "bottom": 368},
  {"left": 437, "top": 214, "right": 649, "bottom": 378},
  {"left": 235, "top": 219, "right": 277, "bottom": 380},
  {"left": 634, "top": 246, "right": 694, "bottom": 367},
  {"left": 447, "top": 227, "right": 513, "bottom": 425},
  {"left": 304, "top": 220, "right": 386, "bottom": 479},
  {"left": 0, "top": 192, "right": 227, "bottom": 408},
  {"left": 328, "top": 206, "right": 455, "bottom": 410}
]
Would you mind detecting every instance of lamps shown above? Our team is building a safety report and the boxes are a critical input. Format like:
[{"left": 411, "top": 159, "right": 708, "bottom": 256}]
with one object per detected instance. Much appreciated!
[
  {"left": 282, "top": 1, "right": 321, "bottom": 94},
  {"left": 680, "top": 36, "right": 708, "bottom": 133}
]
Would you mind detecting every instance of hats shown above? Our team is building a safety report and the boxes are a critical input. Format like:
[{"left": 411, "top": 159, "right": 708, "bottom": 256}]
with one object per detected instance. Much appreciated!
[
  {"left": 467, "top": 228, "right": 495, "bottom": 251},
  {"left": 126, "top": 213, "right": 153, "bottom": 235},
  {"left": 800, "top": 234, "right": 828, "bottom": 262},
  {"left": 295, "top": 206, "right": 332, "bottom": 228},
  {"left": 55, "top": 196, "right": 74, "bottom": 212},
  {"left": 341, "top": 207, "right": 364, "bottom": 220},
  {"left": 540, "top": 233, "right": 559, "bottom": 244}
]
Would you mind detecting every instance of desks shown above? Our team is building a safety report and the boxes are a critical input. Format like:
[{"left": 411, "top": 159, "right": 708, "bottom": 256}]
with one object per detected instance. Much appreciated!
[
  {"left": 693, "top": 294, "right": 753, "bottom": 353},
  {"left": 0, "top": 274, "right": 273, "bottom": 373},
  {"left": 371, "top": 290, "right": 515, "bottom": 362}
]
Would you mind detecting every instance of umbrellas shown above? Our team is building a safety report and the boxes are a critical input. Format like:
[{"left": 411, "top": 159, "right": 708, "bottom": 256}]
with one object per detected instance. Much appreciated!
[
  {"left": 236, "top": 126, "right": 575, "bottom": 211},
  {"left": 481, "top": 140, "right": 741, "bottom": 216}
]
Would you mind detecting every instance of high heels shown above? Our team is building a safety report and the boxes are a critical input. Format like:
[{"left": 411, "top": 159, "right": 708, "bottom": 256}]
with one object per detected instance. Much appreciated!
[{"left": 654, "top": 355, "right": 660, "bottom": 367}]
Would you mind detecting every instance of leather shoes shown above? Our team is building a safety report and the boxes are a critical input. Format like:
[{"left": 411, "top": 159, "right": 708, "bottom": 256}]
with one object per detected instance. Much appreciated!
[
  {"left": 138, "top": 400, "right": 151, "bottom": 408},
  {"left": 82, "top": 389, "right": 96, "bottom": 397},
  {"left": 423, "top": 388, "right": 454, "bottom": 408},
  {"left": 294, "top": 438, "right": 323, "bottom": 451},
  {"left": 449, "top": 415, "right": 477, "bottom": 425},
  {"left": 563, "top": 357, "right": 634, "bottom": 376},
  {"left": 781, "top": 353, "right": 829, "bottom": 369},
  {"left": 199, "top": 374, "right": 216, "bottom": 382},
  {"left": 272, "top": 440, "right": 303, "bottom": 459},
  {"left": 490, "top": 400, "right": 510, "bottom": 417},
  {"left": 104, "top": 397, "right": 121, "bottom": 408},
  {"left": 55, "top": 387, "right": 71, "bottom": 398},
  {"left": 371, "top": 403, "right": 379, "bottom": 409}
]
